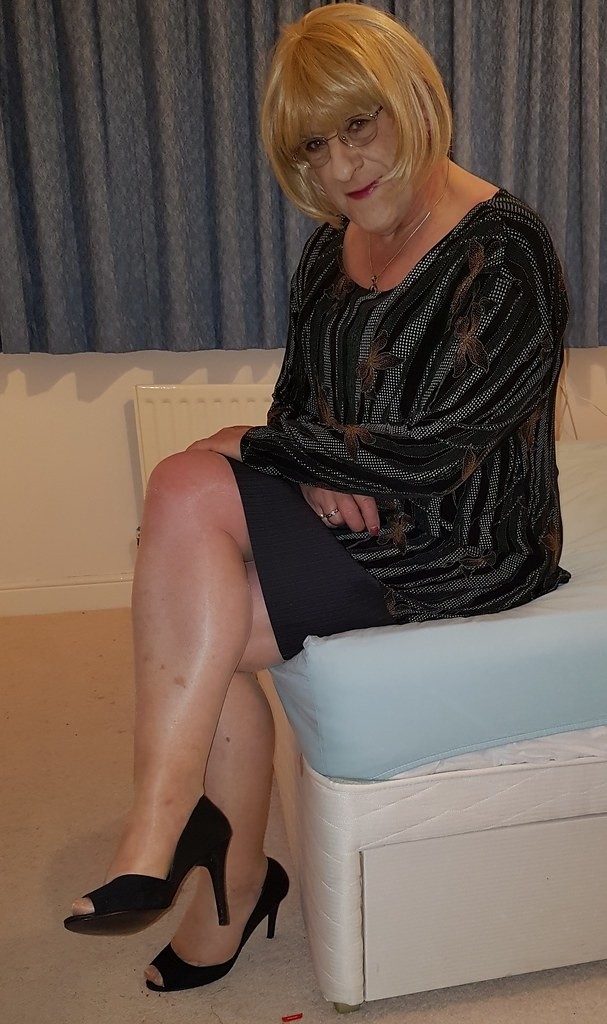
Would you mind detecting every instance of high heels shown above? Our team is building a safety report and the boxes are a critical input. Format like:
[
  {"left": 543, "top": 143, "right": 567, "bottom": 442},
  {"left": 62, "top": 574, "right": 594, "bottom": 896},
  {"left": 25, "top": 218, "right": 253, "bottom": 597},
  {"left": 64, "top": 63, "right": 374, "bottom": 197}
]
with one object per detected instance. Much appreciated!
[
  {"left": 146, "top": 856, "right": 290, "bottom": 992},
  {"left": 64, "top": 794, "right": 231, "bottom": 936}
]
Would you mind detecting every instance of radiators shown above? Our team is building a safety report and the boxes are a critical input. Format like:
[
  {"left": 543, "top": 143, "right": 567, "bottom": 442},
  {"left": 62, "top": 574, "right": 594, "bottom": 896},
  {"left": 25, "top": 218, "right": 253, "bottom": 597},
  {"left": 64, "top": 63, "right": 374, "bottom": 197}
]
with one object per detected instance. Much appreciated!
[{"left": 134, "top": 384, "right": 277, "bottom": 500}]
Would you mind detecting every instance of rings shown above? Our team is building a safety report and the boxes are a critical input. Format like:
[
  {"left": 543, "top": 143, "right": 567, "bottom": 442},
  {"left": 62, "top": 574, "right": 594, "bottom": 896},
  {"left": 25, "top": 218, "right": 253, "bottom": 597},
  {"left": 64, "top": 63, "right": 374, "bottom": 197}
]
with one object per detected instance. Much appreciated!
[
  {"left": 320, "top": 513, "right": 327, "bottom": 519},
  {"left": 325, "top": 509, "right": 339, "bottom": 518}
]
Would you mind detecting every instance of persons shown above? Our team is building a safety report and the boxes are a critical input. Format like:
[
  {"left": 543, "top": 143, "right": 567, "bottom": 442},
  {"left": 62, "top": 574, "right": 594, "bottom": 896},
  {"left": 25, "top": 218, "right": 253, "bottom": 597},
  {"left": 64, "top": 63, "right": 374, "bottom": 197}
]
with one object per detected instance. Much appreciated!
[{"left": 64, "top": 4, "right": 570, "bottom": 991}]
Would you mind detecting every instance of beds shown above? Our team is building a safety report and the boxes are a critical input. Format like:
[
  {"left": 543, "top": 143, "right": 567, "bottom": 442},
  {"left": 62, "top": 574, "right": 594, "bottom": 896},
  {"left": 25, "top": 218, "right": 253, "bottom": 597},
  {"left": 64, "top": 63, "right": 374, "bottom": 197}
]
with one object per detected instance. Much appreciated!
[{"left": 256, "top": 439, "right": 607, "bottom": 1013}]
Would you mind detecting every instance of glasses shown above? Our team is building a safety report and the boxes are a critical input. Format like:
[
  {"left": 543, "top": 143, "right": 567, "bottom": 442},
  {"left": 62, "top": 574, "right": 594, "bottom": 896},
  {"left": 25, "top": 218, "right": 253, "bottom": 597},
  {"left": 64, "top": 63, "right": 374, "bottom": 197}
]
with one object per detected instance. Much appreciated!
[{"left": 292, "top": 105, "right": 383, "bottom": 169}]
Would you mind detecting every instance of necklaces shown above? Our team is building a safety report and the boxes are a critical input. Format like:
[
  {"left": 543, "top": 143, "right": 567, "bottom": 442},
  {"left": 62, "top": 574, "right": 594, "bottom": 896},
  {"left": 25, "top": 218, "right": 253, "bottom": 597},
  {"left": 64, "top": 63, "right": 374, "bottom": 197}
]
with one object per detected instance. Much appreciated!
[{"left": 369, "top": 158, "right": 450, "bottom": 292}]
